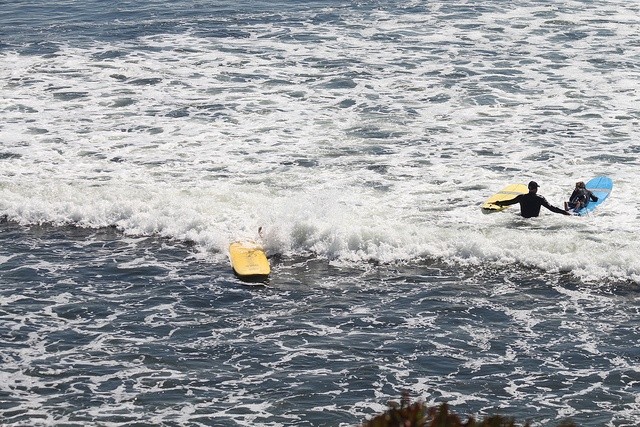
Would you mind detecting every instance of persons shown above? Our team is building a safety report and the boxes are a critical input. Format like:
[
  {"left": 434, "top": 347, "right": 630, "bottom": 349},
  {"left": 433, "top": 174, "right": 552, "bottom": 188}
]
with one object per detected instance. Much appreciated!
[
  {"left": 488, "top": 182, "right": 575, "bottom": 219},
  {"left": 563, "top": 180, "right": 598, "bottom": 215}
]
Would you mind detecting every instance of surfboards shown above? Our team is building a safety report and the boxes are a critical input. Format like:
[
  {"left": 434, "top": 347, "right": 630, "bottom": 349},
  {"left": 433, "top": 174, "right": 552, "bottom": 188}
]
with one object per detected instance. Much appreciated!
[
  {"left": 564, "top": 176, "right": 612, "bottom": 215},
  {"left": 479, "top": 183, "right": 529, "bottom": 210},
  {"left": 229, "top": 240, "right": 270, "bottom": 275}
]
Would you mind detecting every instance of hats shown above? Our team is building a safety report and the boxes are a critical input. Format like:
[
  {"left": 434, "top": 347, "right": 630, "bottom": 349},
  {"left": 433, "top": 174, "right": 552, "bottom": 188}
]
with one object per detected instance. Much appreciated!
[{"left": 528, "top": 181, "right": 540, "bottom": 189}]
